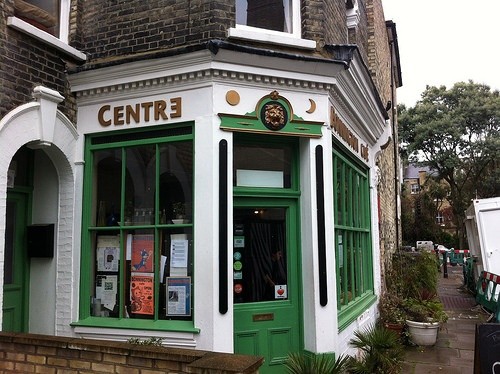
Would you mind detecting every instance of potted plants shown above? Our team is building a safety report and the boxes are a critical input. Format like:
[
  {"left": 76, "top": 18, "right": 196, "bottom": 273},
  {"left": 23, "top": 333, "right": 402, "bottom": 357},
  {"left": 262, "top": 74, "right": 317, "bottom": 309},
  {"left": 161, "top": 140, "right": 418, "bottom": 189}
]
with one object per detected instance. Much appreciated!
[{"left": 379, "top": 248, "right": 451, "bottom": 345}]
